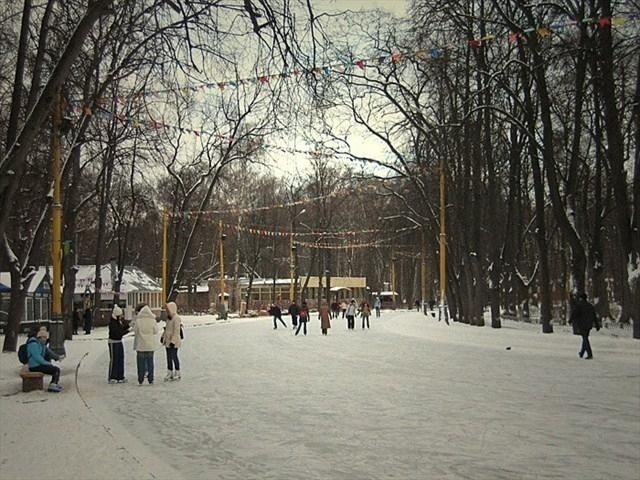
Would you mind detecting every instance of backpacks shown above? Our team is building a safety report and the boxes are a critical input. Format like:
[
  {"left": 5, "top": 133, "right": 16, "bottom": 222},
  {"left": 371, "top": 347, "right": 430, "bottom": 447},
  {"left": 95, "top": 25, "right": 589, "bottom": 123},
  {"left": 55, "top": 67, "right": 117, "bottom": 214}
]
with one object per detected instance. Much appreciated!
[{"left": 18, "top": 337, "right": 38, "bottom": 364}]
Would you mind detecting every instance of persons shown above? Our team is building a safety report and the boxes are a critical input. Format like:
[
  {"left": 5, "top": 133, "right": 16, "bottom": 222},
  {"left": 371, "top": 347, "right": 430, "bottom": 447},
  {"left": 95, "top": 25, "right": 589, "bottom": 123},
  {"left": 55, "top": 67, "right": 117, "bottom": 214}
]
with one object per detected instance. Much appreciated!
[
  {"left": 568, "top": 292, "right": 600, "bottom": 359},
  {"left": 270, "top": 295, "right": 435, "bottom": 335},
  {"left": 27, "top": 302, "right": 183, "bottom": 393}
]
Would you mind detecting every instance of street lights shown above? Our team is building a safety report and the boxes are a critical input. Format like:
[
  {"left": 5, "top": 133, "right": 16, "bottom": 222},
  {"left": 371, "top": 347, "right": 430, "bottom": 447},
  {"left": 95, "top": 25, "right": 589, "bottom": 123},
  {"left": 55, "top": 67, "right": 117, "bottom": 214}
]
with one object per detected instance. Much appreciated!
[
  {"left": 157, "top": 153, "right": 449, "bottom": 323},
  {"left": 48, "top": 87, "right": 74, "bottom": 357}
]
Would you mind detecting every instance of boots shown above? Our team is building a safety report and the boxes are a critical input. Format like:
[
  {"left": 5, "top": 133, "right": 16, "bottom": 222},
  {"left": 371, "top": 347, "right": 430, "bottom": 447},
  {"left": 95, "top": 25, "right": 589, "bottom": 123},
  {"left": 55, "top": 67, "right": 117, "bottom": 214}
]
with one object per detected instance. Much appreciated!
[
  {"left": 165, "top": 369, "right": 172, "bottom": 380},
  {"left": 172, "top": 369, "right": 181, "bottom": 380}
]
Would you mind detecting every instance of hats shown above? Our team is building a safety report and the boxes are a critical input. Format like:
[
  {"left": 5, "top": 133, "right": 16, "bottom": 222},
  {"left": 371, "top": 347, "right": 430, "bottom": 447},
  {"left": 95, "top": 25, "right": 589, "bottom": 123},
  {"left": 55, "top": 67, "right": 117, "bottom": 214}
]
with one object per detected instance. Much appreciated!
[
  {"left": 113, "top": 304, "right": 122, "bottom": 316},
  {"left": 36, "top": 326, "right": 49, "bottom": 339}
]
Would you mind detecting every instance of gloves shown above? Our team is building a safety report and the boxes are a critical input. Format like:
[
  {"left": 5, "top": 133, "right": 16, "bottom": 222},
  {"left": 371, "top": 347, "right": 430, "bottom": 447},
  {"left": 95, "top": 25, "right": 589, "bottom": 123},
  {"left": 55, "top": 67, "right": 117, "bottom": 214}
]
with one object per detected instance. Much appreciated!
[
  {"left": 170, "top": 343, "right": 175, "bottom": 349},
  {"left": 161, "top": 337, "right": 164, "bottom": 343}
]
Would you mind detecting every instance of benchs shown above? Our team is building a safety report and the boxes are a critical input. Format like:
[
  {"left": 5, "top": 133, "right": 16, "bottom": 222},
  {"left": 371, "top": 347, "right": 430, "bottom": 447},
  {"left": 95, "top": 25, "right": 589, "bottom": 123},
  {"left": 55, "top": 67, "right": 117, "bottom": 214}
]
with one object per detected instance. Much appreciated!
[{"left": 19, "top": 366, "right": 44, "bottom": 392}]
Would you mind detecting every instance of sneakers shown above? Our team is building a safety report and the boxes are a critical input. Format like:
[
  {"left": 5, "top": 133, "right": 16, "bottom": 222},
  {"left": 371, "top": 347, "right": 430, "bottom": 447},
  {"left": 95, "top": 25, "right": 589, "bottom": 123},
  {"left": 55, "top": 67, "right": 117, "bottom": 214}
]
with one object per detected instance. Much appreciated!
[
  {"left": 119, "top": 378, "right": 128, "bottom": 383},
  {"left": 57, "top": 384, "right": 64, "bottom": 390},
  {"left": 48, "top": 383, "right": 62, "bottom": 392},
  {"left": 108, "top": 379, "right": 118, "bottom": 384}
]
